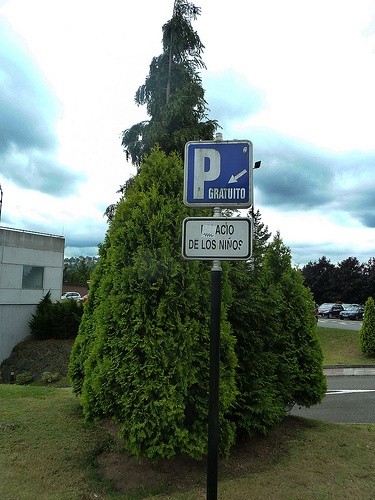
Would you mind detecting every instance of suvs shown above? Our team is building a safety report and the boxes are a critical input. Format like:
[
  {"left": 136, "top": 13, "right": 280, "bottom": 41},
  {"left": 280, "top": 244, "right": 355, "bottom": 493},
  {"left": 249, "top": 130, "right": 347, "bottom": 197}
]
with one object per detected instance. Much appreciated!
[{"left": 317, "top": 303, "right": 343, "bottom": 319}]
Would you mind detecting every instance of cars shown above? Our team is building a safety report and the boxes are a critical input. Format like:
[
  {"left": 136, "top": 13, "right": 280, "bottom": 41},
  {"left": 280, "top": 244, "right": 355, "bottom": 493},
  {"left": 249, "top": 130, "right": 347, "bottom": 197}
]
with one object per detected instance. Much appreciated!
[
  {"left": 339, "top": 306, "right": 363, "bottom": 320},
  {"left": 342, "top": 304, "right": 364, "bottom": 310},
  {"left": 62, "top": 292, "right": 81, "bottom": 300}
]
